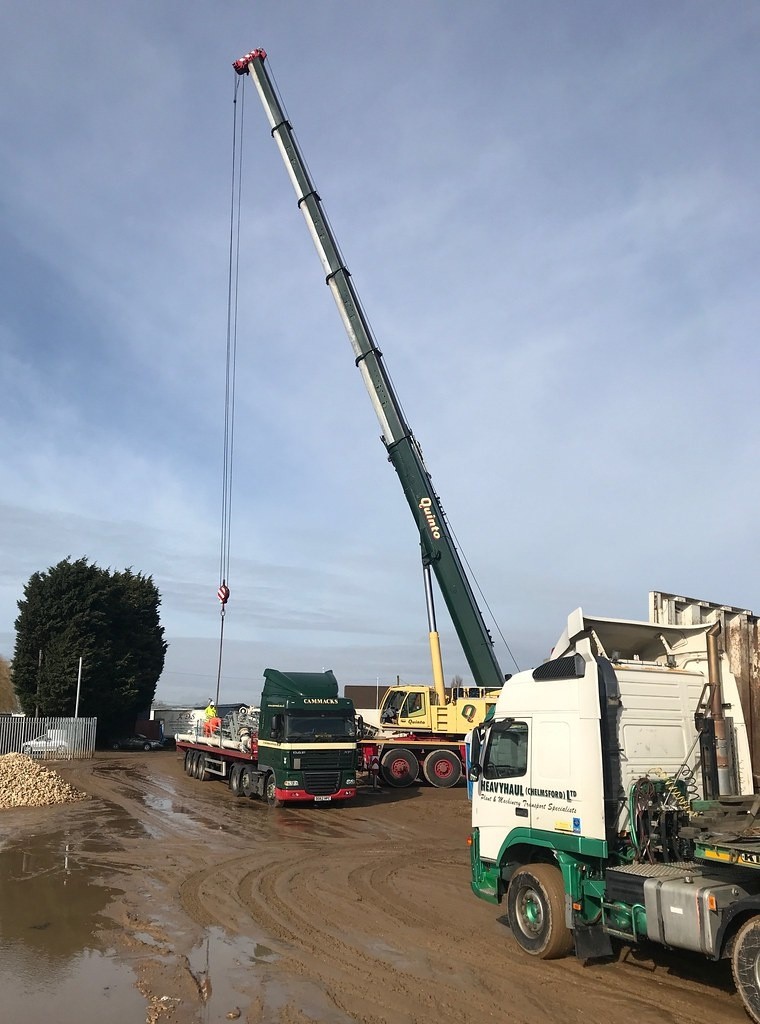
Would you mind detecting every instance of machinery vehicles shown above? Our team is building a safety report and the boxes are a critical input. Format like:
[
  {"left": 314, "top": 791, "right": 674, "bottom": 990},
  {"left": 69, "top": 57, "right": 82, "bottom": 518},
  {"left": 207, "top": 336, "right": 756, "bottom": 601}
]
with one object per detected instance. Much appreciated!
[{"left": 206, "top": 44, "right": 511, "bottom": 784}]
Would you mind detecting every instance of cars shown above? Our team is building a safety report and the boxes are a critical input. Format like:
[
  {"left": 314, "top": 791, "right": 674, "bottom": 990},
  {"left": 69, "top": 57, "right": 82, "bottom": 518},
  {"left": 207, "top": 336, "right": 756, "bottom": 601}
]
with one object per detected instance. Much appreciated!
[
  {"left": 107, "top": 733, "right": 162, "bottom": 751},
  {"left": 23, "top": 732, "right": 69, "bottom": 753}
]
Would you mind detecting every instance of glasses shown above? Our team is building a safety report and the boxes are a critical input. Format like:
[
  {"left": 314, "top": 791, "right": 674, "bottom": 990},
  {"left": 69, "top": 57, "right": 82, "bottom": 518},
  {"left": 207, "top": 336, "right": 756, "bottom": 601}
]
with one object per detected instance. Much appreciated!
[{"left": 211, "top": 705, "right": 214, "bottom": 707}]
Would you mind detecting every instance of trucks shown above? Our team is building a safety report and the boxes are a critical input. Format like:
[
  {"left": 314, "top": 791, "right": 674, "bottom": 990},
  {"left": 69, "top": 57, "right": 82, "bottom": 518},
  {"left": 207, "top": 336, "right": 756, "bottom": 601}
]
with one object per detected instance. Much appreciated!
[{"left": 461, "top": 600, "right": 759, "bottom": 1019}]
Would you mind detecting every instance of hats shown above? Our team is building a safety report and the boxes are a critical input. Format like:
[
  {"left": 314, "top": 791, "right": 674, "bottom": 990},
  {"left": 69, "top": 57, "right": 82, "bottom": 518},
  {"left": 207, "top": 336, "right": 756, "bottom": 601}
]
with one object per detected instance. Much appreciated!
[{"left": 210, "top": 701, "right": 214, "bottom": 705}]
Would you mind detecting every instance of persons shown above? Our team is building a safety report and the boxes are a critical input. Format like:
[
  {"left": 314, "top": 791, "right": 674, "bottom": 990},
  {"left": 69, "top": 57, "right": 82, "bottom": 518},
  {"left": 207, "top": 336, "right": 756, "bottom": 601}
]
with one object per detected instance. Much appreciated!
[{"left": 204, "top": 702, "right": 222, "bottom": 737}]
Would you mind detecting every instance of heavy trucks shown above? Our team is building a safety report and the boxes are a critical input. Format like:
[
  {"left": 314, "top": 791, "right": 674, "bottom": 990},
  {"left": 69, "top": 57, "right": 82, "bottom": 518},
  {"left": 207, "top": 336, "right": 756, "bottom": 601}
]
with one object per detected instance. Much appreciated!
[{"left": 172, "top": 667, "right": 363, "bottom": 811}]
